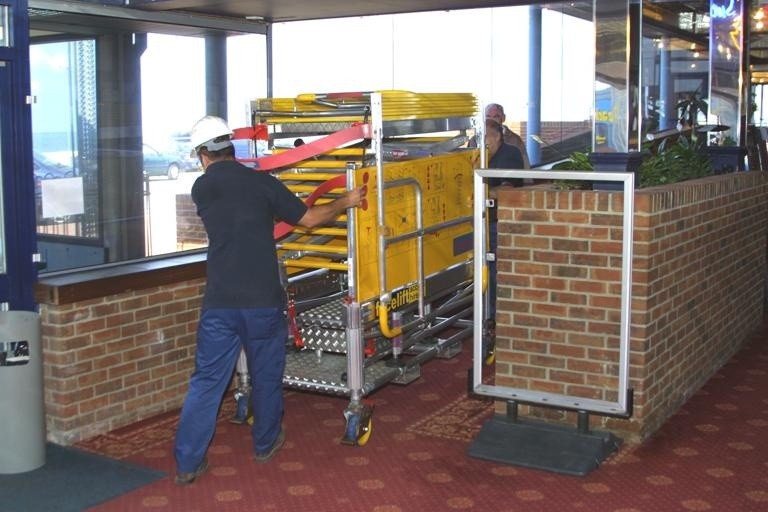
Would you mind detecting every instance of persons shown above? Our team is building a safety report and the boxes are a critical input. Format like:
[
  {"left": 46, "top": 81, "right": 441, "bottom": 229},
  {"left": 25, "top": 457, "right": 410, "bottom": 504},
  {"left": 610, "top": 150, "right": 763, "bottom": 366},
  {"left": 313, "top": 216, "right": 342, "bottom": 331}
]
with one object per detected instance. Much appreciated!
[
  {"left": 484, "top": 120, "right": 525, "bottom": 332},
  {"left": 484, "top": 104, "right": 533, "bottom": 185},
  {"left": 172, "top": 116, "right": 367, "bottom": 484}
]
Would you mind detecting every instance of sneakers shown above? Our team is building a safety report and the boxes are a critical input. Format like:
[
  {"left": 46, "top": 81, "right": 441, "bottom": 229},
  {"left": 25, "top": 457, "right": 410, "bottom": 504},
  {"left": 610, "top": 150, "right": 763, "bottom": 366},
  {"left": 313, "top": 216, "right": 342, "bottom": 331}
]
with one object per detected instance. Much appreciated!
[
  {"left": 176, "top": 456, "right": 207, "bottom": 484},
  {"left": 255, "top": 428, "right": 285, "bottom": 460}
]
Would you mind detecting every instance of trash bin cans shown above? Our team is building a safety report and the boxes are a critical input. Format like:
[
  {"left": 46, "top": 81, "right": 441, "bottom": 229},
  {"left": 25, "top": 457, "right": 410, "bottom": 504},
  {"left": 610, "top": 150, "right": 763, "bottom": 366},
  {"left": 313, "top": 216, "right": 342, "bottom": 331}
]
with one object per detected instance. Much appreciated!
[{"left": 0, "top": 310, "right": 46, "bottom": 475}]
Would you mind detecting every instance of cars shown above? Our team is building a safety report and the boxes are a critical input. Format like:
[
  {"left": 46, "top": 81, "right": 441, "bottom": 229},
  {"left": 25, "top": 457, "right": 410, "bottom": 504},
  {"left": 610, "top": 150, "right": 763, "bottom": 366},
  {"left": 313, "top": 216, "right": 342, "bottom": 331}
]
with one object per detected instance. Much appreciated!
[{"left": 141, "top": 140, "right": 180, "bottom": 180}]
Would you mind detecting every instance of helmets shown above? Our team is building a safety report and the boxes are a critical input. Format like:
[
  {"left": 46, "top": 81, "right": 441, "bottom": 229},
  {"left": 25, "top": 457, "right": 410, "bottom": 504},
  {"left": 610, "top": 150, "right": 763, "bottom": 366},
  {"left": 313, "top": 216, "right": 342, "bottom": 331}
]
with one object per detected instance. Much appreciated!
[{"left": 189, "top": 115, "right": 235, "bottom": 157}]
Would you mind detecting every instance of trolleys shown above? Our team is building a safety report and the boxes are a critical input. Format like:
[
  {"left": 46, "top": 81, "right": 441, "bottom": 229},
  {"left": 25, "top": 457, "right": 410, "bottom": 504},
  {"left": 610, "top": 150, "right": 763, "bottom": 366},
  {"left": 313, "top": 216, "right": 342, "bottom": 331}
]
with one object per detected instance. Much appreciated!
[{"left": 234, "top": 141, "right": 495, "bottom": 448}]
